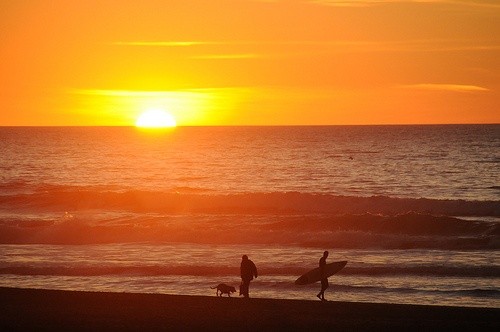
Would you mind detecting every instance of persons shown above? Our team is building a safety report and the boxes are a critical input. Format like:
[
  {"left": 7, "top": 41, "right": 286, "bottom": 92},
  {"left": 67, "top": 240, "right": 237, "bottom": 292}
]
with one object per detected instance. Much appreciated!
[
  {"left": 317, "top": 250, "right": 330, "bottom": 301},
  {"left": 239, "top": 254, "right": 257, "bottom": 298}
]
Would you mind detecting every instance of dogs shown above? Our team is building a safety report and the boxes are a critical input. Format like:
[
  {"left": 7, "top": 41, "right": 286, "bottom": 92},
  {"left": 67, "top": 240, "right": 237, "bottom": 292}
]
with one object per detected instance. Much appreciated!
[{"left": 210, "top": 284, "right": 236, "bottom": 297}]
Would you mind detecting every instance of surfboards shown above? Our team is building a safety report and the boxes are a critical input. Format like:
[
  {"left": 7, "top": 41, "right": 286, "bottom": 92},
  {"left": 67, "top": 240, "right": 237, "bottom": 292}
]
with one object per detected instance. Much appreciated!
[{"left": 295, "top": 260, "right": 347, "bottom": 284}]
